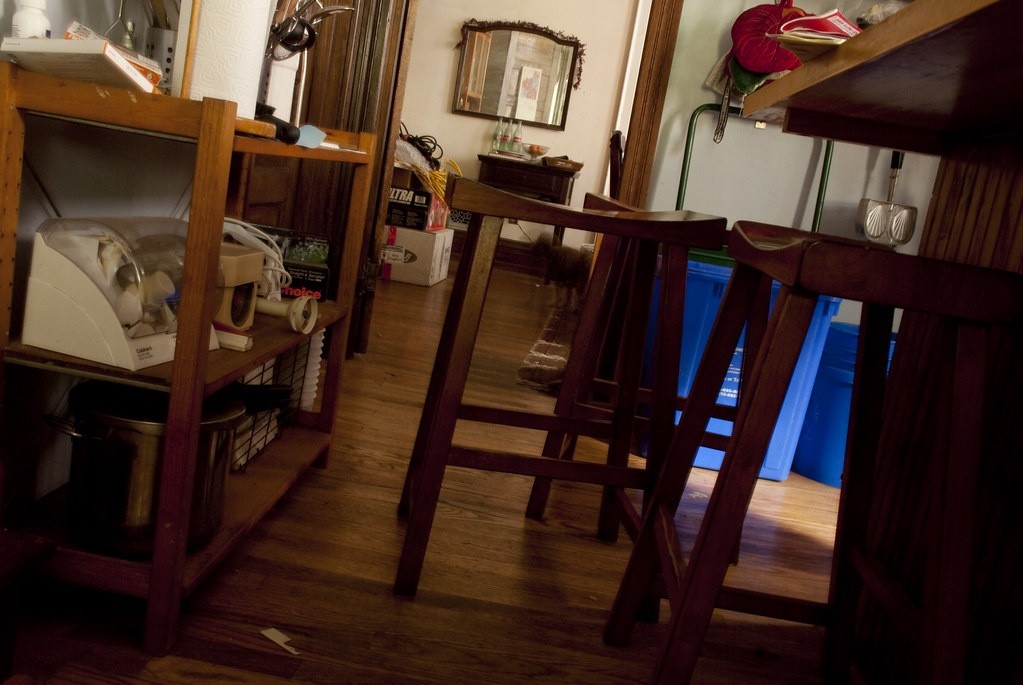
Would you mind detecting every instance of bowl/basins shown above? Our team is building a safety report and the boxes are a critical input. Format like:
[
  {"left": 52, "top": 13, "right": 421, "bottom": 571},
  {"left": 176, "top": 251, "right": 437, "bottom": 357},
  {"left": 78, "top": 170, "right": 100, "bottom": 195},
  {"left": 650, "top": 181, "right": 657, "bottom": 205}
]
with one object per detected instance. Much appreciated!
[{"left": 543, "top": 156, "right": 584, "bottom": 171}]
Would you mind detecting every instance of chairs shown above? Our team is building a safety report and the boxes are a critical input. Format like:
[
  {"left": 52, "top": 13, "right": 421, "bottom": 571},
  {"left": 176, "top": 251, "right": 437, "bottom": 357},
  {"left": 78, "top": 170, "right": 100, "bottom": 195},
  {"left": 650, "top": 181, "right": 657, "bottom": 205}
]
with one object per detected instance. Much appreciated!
[{"left": 610, "top": 131, "right": 624, "bottom": 197}]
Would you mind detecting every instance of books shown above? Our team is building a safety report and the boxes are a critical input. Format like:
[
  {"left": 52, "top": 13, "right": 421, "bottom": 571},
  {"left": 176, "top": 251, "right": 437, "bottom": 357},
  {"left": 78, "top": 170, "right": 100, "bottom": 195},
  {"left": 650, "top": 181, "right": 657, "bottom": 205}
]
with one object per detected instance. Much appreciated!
[
  {"left": 764, "top": 8, "right": 864, "bottom": 65},
  {"left": 0, "top": 20, "right": 163, "bottom": 95}
]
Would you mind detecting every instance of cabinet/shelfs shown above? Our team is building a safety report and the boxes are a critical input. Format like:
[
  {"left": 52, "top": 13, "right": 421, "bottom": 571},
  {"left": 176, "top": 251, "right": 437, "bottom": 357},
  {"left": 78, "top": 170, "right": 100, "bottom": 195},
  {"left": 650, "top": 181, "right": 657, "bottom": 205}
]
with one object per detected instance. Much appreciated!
[
  {"left": 460, "top": 30, "right": 492, "bottom": 113},
  {"left": 0, "top": 63, "right": 377, "bottom": 659}
]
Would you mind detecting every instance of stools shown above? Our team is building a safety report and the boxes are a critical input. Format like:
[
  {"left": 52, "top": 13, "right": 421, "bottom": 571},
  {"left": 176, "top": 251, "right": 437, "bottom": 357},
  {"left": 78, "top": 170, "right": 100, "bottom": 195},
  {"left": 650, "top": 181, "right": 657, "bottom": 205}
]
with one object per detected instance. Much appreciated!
[
  {"left": 393, "top": 170, "right": 728, "bottom": 622},
  {"left": 524, "top": 191, "right": 775, "bottom": 565},
  {"left": 603, "top": 221, "right": 1022, "bottom": 684}
]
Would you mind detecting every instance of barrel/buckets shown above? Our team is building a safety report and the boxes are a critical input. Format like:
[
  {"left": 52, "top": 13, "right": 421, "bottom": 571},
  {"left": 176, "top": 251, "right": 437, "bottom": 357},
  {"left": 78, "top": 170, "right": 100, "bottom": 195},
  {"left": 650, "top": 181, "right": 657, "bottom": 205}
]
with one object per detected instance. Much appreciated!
[{"left": 791, "top": 320, "right": 898, "bottom": 486}]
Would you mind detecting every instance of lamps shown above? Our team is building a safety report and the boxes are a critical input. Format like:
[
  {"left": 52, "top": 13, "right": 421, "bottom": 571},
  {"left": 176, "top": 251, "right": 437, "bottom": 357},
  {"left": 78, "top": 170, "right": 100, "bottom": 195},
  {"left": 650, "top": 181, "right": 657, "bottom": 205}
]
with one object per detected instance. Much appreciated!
[{"left": 857, "top": 0, "right": 918, "bottom": 246}]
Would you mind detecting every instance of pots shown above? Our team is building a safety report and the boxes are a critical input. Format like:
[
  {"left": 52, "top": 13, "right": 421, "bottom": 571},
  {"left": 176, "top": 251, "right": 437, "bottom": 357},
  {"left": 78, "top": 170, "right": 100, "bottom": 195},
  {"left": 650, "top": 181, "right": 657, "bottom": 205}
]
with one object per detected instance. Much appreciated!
[{"left": 45, "top": 382, "right": 245, "bottom": 555}]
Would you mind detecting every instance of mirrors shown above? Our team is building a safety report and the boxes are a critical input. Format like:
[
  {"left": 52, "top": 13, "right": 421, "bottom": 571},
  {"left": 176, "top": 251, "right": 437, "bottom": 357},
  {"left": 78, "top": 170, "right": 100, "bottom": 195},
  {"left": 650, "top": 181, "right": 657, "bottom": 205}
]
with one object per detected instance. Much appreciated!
[{"left": 452, "top": 25, "right": 579, "bottom": 131}]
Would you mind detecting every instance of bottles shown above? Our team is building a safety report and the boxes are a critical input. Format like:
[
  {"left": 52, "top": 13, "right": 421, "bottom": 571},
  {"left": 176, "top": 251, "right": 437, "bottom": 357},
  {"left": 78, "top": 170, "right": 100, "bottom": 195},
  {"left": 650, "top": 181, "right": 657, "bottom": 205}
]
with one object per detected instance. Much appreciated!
[
  {"left": 491, "top": 117, "right": 504, "bottom": 150},
  {"left": 11, "top": 0, "right": 51, "bottom": 39},
  {"left": 512, "top": 120, "right": 522, "bottom": 153},
  {"left": 500, "top": 119, "right": 513, "bottom": 151}
]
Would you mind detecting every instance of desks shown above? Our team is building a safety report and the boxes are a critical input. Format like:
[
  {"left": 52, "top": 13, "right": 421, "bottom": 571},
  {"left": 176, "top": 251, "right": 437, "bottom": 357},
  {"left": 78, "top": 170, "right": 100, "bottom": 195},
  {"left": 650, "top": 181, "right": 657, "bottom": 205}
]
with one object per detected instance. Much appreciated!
[{"left": 478, "top": 154, "right": 581, "bottom": 285}]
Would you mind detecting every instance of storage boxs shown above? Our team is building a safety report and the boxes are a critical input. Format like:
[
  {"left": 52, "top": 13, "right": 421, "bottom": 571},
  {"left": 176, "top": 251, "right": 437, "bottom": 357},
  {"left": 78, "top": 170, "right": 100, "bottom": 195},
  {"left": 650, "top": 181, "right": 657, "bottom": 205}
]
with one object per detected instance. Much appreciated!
[
  {"left": 641, "top": 253, "right": 899, "bottom": 488},
  {"left": 392, "top": 166, "right": 422, "bottom": 188},
  {"left": 249, "top": 224, "right": 329, "bottom": 303},
  {"left": 379, "top": 225, "right": 454, "bottom": 287},
  {"left": 543, "top": 157, "right": 583, "bottom": 172},
  {"left": 211, "top": 242, "right": 264, "bottom": 332},
  {"left": 385, "top": 187, "right": 448, "bottom": 231}
]
